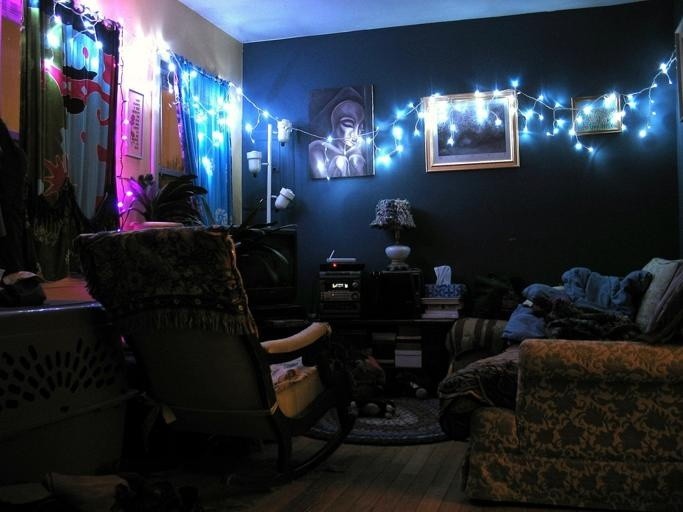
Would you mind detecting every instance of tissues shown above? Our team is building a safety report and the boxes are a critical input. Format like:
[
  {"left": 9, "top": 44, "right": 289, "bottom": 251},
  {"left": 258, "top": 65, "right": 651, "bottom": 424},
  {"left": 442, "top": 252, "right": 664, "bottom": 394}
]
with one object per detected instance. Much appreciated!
[{"left": 424, "top": 265, "right": 466, "bottom": 298}]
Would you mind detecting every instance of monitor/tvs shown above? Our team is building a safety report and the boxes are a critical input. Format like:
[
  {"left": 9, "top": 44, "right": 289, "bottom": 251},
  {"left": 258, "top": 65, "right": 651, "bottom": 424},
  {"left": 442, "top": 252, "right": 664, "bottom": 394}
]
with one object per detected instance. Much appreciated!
[{"left": 361, "top": 270, "right": 423, "bottom": 320}]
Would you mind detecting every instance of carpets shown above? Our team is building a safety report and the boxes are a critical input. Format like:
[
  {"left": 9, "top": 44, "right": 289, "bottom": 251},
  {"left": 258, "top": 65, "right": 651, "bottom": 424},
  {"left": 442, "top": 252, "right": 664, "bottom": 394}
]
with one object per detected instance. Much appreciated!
[{"left": 305, "top": 398, "right": 452, "bottom": 446}]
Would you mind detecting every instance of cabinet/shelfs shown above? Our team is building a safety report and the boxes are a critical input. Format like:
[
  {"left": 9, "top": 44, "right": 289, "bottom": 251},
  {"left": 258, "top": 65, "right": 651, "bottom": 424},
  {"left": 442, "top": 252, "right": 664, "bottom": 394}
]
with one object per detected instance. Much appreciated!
[{"left": 312, "top": 319, "right": 455, "bottom": 395}]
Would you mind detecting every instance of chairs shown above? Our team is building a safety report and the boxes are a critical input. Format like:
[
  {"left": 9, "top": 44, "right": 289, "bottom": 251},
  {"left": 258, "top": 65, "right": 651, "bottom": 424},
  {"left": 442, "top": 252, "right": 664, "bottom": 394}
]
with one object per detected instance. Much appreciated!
[{"left": 77, "top": 221, "right": 356, "bottom": 512}]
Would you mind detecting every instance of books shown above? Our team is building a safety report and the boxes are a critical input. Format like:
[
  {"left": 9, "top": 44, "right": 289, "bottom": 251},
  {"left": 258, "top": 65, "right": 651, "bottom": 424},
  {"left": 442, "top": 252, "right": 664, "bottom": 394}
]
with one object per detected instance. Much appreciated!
[
  {"left": 420, "top": 295, "right": 464, "bottom": 319},
  {"left": 340, "top": 329, "right": 423, "bottom": 370},
  {"left": 38, "top": 277, "right": 96, "bottom": 305}
]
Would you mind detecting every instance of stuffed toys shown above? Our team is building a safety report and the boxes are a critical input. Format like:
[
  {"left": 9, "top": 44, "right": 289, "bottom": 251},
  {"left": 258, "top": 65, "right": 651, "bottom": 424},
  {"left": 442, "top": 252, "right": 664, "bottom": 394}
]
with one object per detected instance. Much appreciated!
[
  {"left": 349, "top": 398, "right": 396, "bottom": 419},
  {"left": 395, "top": 371, "right": 431, "bottom": 398}
]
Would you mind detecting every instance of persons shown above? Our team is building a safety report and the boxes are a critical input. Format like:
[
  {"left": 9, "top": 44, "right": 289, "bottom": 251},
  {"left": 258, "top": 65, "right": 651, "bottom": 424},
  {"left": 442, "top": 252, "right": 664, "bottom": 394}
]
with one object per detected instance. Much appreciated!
[{"left": 306, "top": 100, "right": 369, "bottom": 178}]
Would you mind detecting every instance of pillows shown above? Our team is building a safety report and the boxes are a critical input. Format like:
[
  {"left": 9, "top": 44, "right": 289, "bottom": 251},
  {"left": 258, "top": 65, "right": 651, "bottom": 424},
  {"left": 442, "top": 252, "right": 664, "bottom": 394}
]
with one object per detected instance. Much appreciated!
[{"left": 634, "top": 259, "right": 680, "bottom": 333}]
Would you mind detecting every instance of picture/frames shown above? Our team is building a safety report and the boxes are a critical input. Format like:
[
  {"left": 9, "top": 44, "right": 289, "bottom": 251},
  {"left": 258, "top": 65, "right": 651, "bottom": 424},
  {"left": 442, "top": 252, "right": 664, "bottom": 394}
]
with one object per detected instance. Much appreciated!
[
  {"left": 123, "top": 88, "right": 145, "bottom": 160},
  {"left": 421, "top": 89, "right": 623, "bottom": 174}
]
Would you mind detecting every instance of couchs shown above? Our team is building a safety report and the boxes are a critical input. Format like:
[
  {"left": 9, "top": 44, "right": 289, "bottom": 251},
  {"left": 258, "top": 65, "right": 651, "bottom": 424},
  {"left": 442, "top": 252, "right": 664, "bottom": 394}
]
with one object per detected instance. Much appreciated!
[{"left": 459, "top": 285, "right": 683, "bottom": 511}]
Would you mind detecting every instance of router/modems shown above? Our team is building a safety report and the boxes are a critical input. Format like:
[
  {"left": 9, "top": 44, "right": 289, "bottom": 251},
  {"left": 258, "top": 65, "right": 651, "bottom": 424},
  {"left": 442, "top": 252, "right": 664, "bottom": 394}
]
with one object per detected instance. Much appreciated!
[{"left": 327, "top": 250, "right": 357, "bottom": 265}]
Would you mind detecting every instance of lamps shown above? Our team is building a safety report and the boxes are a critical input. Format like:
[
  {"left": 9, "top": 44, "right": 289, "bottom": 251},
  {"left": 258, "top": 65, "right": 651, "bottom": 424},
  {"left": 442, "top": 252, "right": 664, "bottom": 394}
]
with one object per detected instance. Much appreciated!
[
  {"left": 246, "top": 119, "right": 295, "bottom": 225},
  {"left": 369, "top": 196, "right": 417, "bottom": 271}
]
